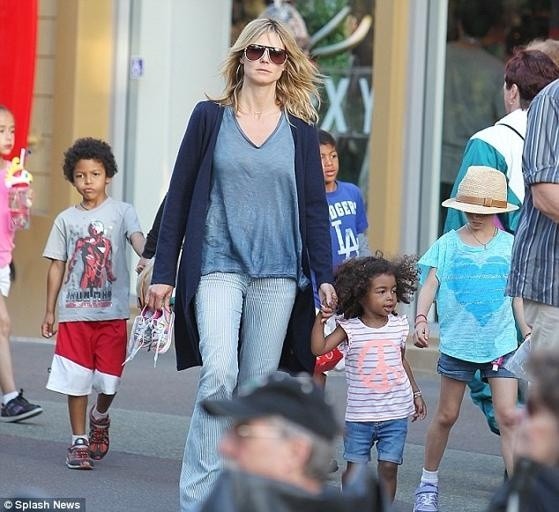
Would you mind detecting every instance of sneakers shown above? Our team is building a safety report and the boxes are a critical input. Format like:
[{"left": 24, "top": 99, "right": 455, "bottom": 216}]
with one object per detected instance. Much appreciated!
[
  {"left": 66, "top": 438, "right": 100, "bottom": 468},
  {"left": 412, "top": 483, "right": 440, "bottom": 511},
  {"left": 1, "top": 396, "right": 44, "bottom": 422},
  {"left": 88, "top": 405, "right": 111, "bottom": 461},
  {"left": 133, "top": 302, "right": 176, "bottom": 355}
]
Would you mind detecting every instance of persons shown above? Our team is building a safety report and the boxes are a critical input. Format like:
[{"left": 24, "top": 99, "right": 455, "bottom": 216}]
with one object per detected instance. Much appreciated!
[
  {"left": 138, "top": 2, "right": 559, "bottom": 510},
  {"left": 42, "top": 139, "right": 146, "bottom": 470},
  {"left": 0, "top": 108, "right": 42, "bottom": 422}
]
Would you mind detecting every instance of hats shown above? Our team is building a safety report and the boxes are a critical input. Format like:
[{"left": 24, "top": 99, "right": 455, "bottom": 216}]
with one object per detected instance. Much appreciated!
[
  {"left": 441, "top": 166, "right": 520, "bottom": 215},
  {"left": 199, "top": 374, "right": 337, "bottom": 441}
]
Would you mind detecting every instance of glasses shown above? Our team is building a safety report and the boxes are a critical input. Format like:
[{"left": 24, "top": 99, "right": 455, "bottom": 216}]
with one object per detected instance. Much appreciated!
[{"left": 245, "top": 44, "right": 288, "bottom": 65}]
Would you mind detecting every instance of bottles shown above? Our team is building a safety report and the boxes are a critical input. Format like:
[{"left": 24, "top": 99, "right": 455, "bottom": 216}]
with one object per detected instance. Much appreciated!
[
  {"left": 491, "top": 355, "right": 503, "bottom": 372},
  {"left": 10, "top": 169, "right": 33, "bottom": 231}
]
